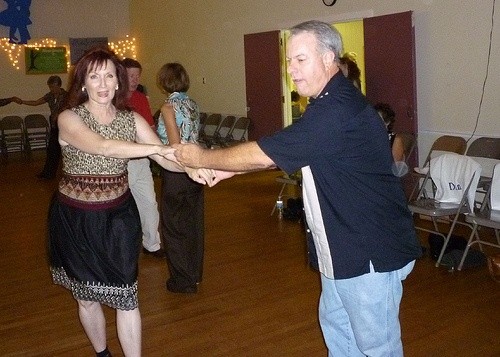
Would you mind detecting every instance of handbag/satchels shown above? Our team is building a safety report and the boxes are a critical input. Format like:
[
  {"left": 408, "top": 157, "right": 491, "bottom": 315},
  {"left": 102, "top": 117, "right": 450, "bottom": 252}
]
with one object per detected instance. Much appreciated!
[{"left": 486, "top": 253, "right": 500, "bottom": 284}]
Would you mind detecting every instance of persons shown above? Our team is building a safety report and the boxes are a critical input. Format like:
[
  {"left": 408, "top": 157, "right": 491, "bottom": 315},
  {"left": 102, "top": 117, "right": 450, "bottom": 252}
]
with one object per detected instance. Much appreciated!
[
  {"left": 0, "top": 98, "right": 23, "bottom": 109},
  {"left": 150, "top": 61, "right": 205, "bottom": 291},
  {"left": 18, "top": 75, "right": 71, "bottom": 178},
  {"left": 47, "top": 44, "right": 215, "bottom": 357},
  {"left": 370, "top": 102, "right": 404, "bottom": 177},
  {"left": 338, "top": 55, "right": 362, "bottom": 92},
  {"left": 118, "top": 57, "right": 168, "bottom": 258},
  {"left": 173, "top": 20, "right": 422, "bottom": 357}
]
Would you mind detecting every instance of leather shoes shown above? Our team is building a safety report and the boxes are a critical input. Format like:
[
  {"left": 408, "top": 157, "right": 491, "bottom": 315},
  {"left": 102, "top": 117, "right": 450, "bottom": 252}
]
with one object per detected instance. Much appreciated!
[{"left": 142, "top": 248, "right": 167, "bottom": 257}]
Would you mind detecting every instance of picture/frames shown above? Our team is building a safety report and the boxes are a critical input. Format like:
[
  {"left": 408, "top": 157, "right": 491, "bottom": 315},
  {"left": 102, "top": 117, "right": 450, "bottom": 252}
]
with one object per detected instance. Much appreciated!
[
  {"left": 24, "top": 46, "right": 68, "bottom": 74},
  {"left": 69, "top": 37, "right": 110, "bottom": 66}
]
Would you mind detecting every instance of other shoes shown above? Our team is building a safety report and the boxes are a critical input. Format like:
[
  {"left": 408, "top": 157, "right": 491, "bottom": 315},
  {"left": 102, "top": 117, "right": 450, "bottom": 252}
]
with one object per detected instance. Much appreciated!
[{"left": 167, "top": 277, "right": 202, "bottom": 292}]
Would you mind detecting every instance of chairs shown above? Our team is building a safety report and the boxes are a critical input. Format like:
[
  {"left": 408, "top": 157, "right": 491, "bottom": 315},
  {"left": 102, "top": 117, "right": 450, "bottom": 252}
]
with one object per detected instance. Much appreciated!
[
  {"left": 210, "top": 116, "right": 251, "bottom": 149},
  {"left": 456, "top": 203, "right": 500, "bottom": 274},
  {"left": 406, "top": 135, "right": 500, "bottom": 269},
  {"left": 24, "top": 113, "right": 50, "bottom": 159},
  {"left": 197, "top": 113, "right": 224, "bottom": 149},
  {"left": 202, "top": 115, "right": 236, "bottom": 150},
  {"left": 403, "top": 134, "right": 469, "bottom": 232},
  {"left": 267, "top": 171, "right": 304, "bottom": 218},
  {"left": 195, "top": 113, "right": 208, "bottom": 139},
  {"left": 1, "top": 115, "right": 30, "bottom": 159}
]
